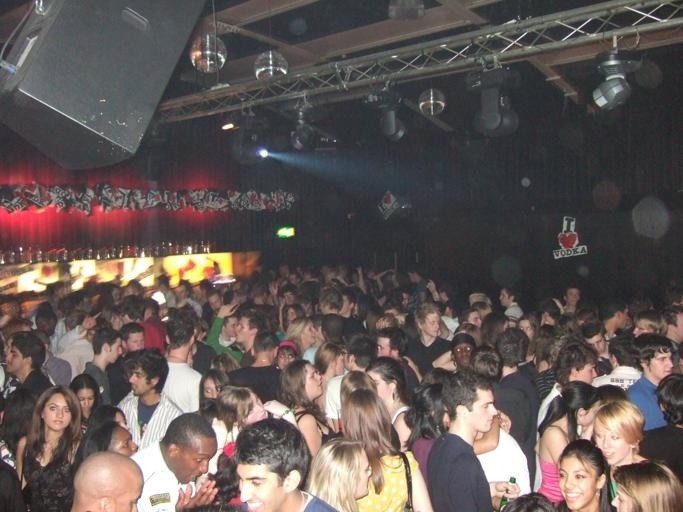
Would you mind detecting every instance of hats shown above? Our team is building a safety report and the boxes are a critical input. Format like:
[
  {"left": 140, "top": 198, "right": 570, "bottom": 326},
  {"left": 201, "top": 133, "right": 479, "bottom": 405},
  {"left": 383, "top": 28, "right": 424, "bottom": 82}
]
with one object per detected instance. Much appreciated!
[
  {"left": 467, "top": 291, "right": 493, "bottom": 310},
  {"left": 278, "top": 340, "right": 299, "bottom": 354},
  {"left": 450, "top": 333, "right": 477, "bottom": 349}
]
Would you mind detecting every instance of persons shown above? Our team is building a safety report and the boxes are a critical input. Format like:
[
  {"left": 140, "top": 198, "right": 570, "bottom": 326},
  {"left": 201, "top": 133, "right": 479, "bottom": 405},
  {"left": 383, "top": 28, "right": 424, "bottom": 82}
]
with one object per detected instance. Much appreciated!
[
  {"left": 365, "top": 356, "right": 421, "bottom": 452},
  {"left": 340, "top": 387, "right": 433, "bottom": 512},
  {"left": 450, "top": 333, "right": 477, "bottom": 375},
  {"left": 404, "top": 301, "right": 453, "bottom": 378},
  {"left": 611, "top": 462, "right": 683, "bottom": 512},
  {"left": 74, "top": 420, "right": 138, "bottom": 468},
  {"left": 0, "top": 331, "right": 54, "bottom": 460},
  {"left": 82, "top": 327, "right": 123, "bottom": 412},
  {"left": 215, "top": 384, "right": 300, "bottom": 448},
  {"left": 626, "top": 333, "right": 674, "bottom": 432},
  {"left": 70, "top": 451, "right": 143, "bottom": 512},
  {"left": 29, "top": 328, "right": 72, "bottom": 387},
  {"left": 304, "top": 436, "right": 372, "bottom": 512},
  {"left": 340, "top": 370, "right": 378, "bottom": 409},
  {"left": 427, "top": 368, "right": 498, "bottom": 512},
  {"left": 275, "top": 359, "right": 338, "bottom": 458},
  {"left": 16, "top": 385, "right": 84, "bottom": 512},
  {"left": 591, "top": 334, "right": 643, "bottom": 392},
  {"left": 87, "top": 405, "right": 129, "bottom": 430},
  {"left": 493, "top": 327, "right": 541, "bottom": 492},
  {"left": 324, "top": 333, "right": 379, "bottom": 433},
  {"left": 232, "top": 418, "right": 338, "bottom": 512},
  {"left": 0, "top": 395, "right": 27, "bottom": 512},
  {"left": 198, "top": 369, "right": 232, "bottom": 399},
  {"left": 70, "top": 374, "right": 103, "bottom": 437},
  {"left": 159, "top": 306, "right": 202, "bottom": 413},
  {"left": 0, "top": 274, "right": 207, "bottom": 343},
  {"left": 637, "top": 373, "right": 683, "bottom": 486},
  {"left": 231, "top": 331, "right": 281, "bottom": 404},
  {"left": 533, "top": 339, "right": 597, "bottom": 494},
  {"left": 469, "top": 345, "right": 504, "bottom": 382},
  {"left": 105, "top": 322, "right": 145, "bottom": 405},
  {"left": 403, "top": 382, "right": 512, "bottom": 491},
  {"left": 593, "top": 400, "right": 675, "bottom": 512},
  {"left": 555, "top": 438, "right": 616, "bottom": 512},
  {"left": 128, "top": 413, "right": 218, "bottom": 512},
  {"left": 377, "top": 326, "right": 420, "bottom": 388},
  {"left": 537, "top": 380, "right": 607, "bottom": 509},
  {"left": 206, "top": 263, "right": 682, "bottom": 342},
  {"left": 116, "top": 348, "right": 184, "bottom": 452}
]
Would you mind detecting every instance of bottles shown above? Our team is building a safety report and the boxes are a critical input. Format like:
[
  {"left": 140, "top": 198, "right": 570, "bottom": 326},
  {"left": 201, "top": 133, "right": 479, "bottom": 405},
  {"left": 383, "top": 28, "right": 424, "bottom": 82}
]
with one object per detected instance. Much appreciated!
[
  {"left": 500, "top": 477, "right": 516, "bottom": 512},
  {"left": 1, "top": 241, "right": 218, "bottom": 264}
]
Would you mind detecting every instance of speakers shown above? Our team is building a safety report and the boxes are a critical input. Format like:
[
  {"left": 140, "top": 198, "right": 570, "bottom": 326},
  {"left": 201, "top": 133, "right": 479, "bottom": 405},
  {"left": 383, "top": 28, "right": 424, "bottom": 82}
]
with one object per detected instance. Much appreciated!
[{"left": 2, "top": 0, "right": 207, "bottom": 172}]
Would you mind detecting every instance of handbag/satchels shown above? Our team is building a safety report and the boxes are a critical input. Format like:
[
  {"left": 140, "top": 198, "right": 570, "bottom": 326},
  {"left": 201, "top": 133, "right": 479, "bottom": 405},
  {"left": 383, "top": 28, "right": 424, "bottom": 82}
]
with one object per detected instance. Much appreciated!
[{"left": 399, "top": 451, "right": 414, "bottom": 512}]
